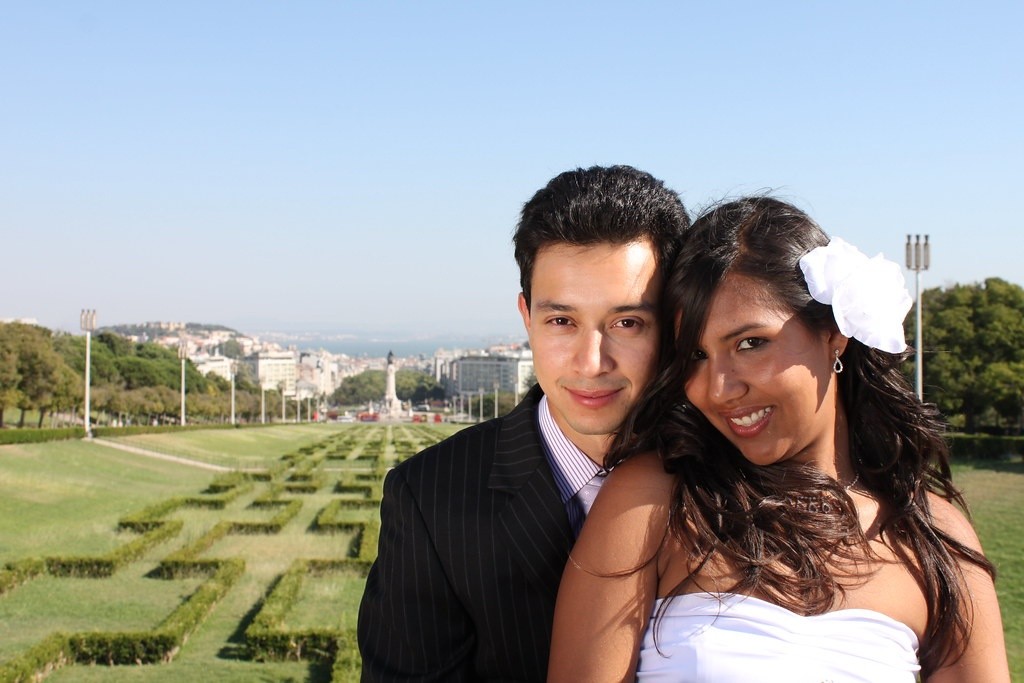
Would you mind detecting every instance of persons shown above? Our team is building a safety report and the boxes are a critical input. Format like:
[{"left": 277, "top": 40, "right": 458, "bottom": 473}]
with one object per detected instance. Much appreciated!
[{"left": 357, "top": 166, "right": 1012, "bottom": 682}]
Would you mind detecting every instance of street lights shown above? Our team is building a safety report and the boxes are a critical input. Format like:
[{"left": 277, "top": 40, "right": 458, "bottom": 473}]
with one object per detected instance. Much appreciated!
[
  {"left": 229, "top": 364, "right": 239, "bottom": 423},
  {"left": 176, "top": 345, "right": 188, "bottom": 424},
  {"left": 904, "top": 233, "right": 932, "bottom": 402},
  {"left": 259, "top": 378, "right": 321, "bottom": 425},
  {"left": 79, "top": 308, "right": 96, "bottom": 437}
]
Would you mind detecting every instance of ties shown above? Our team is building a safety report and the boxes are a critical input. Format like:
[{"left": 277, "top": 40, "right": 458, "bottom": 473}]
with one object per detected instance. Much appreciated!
[{"left": 577, "top": 475, "right": 606, "bottom": 517}]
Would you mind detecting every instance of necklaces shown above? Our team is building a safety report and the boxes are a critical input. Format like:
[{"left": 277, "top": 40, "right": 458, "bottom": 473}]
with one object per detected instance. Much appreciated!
[{"left": 789, "top": 472, "right": 859, "bottom": 514}]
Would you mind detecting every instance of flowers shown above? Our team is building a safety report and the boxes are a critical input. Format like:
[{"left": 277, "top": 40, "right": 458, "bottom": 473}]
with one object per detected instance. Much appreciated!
[{"left": 799, "top": 229, "right": 918, "bottom": 353}]
